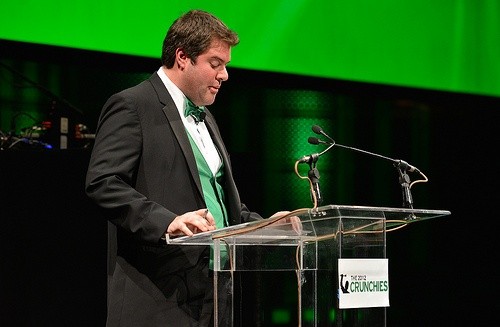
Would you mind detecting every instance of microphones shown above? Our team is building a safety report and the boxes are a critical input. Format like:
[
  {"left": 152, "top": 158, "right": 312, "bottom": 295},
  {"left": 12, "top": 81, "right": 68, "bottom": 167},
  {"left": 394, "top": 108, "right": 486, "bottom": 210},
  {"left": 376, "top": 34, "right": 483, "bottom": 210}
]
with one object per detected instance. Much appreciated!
[
  {"left": 307, "top": 137, "right": 422, "bottom": 175},
  {"left": 297, "top": 125, "right": 336, "bottom": 165}
]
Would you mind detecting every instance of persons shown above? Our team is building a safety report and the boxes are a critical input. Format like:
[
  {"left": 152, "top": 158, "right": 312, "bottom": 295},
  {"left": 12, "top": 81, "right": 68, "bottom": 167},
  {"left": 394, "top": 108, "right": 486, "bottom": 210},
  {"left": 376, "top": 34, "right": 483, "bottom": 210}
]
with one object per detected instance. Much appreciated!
[{"left": 85, "top": 6, "right": 303, "bottom": 327}]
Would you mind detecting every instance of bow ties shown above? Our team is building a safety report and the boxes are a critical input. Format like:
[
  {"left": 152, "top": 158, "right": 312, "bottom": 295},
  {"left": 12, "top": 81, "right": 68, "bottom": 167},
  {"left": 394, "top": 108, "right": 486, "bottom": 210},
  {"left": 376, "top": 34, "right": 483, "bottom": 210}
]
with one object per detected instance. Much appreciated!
[{"left": 184, "top": 98, "right": 205, "bottom": 121}]
